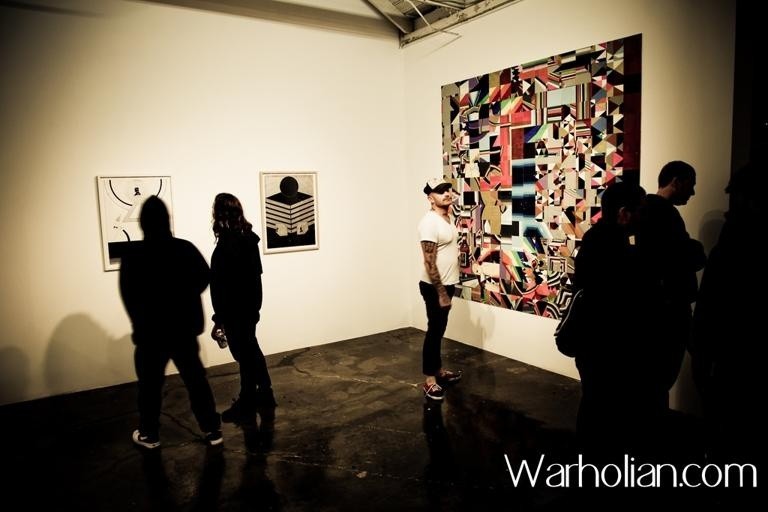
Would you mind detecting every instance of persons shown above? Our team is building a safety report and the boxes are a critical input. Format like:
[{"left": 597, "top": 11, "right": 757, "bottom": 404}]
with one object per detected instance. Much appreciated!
[
  {"left": 630, "top": 160, "right": 709, "bottom": 465},
  {"left": 209, "top": 192, "right": 277, "bottom": 424},
  {"left": 415, "top": 177, "right": 466, "bottom": 401},
  {"left": 572, "top": 184, "right": 649, "bottom": 458},
  {"left": 119, "top": 195, "right": 225, "bottom": 451}
]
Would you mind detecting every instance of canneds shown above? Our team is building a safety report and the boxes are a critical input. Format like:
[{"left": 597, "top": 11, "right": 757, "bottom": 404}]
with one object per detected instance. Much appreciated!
[{"left": 216, "top": 329, "right": 229, "bottom": 348}]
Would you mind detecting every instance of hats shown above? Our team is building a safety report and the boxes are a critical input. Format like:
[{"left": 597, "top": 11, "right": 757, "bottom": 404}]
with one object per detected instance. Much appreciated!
[{"left": 423, "top": 178, "right": 452, "bottom": 195}]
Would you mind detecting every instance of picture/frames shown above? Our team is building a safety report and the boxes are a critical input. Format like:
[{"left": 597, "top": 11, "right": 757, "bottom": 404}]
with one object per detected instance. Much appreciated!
[
  {"left": 258, "top": 170, "right": 320, "bottom": 255},
  {"left": 95, "top": 175, "right": 174, "bottom": 273}
]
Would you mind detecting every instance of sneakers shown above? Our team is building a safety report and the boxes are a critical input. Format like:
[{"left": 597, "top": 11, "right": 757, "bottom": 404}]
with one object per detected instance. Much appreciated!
[
  {"left": 440, "top": 368, "right": 463, "bottom": 381},
  {"left": 222, "top": 401, "right": 248, "bottom": 421},
  {"left": 424, "top": 383, "right": 444, "bottom": 400},
  {"left": 133, "top": 430, "right": 160, "bottom": 449},
  {"left": 198, "top": 433, "right": 224, "bottom": 450},
  {"left": 256, "top": 396, "right": 278, "bottom": 411}
]
